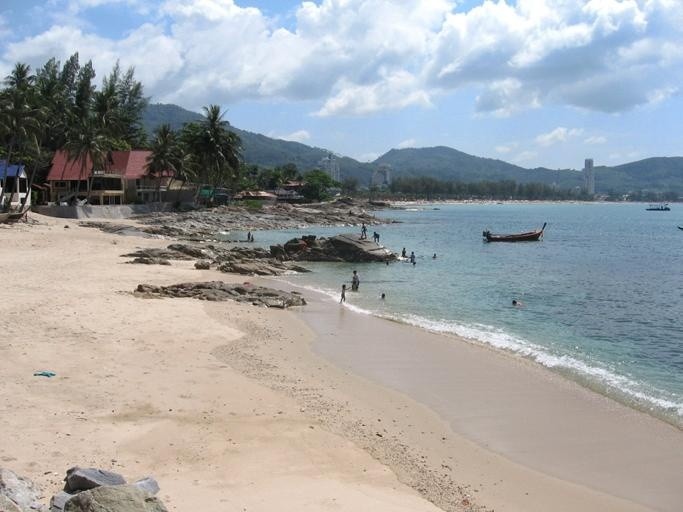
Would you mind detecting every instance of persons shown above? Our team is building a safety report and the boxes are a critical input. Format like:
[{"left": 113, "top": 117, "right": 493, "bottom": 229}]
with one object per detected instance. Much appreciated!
[
  {"left": 512, "top": 300, "right": 522, "bottom": 306},
  {"left": 340, "top": 222, "right": 417, "bottom": 302},
  {"left": 432, "top": 254, "right": 436, "bottom": 258},
  {"left": 248, "top": 232, "right": 251, "bottom": 241},
  {"left": 251, "top": 235, "right": 254, "bottom": 242}
]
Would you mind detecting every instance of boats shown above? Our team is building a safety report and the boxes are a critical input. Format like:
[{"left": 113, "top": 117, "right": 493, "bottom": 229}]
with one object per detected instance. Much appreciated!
[
  {"left": 483, "top": 222, "right": 546, "bottom": 243},
  {"left": 646, "top": 206, "right": 670, "bottom": 211},
  {"left": 0, "top": 206, "right": 30, "bottom": 223}
]
